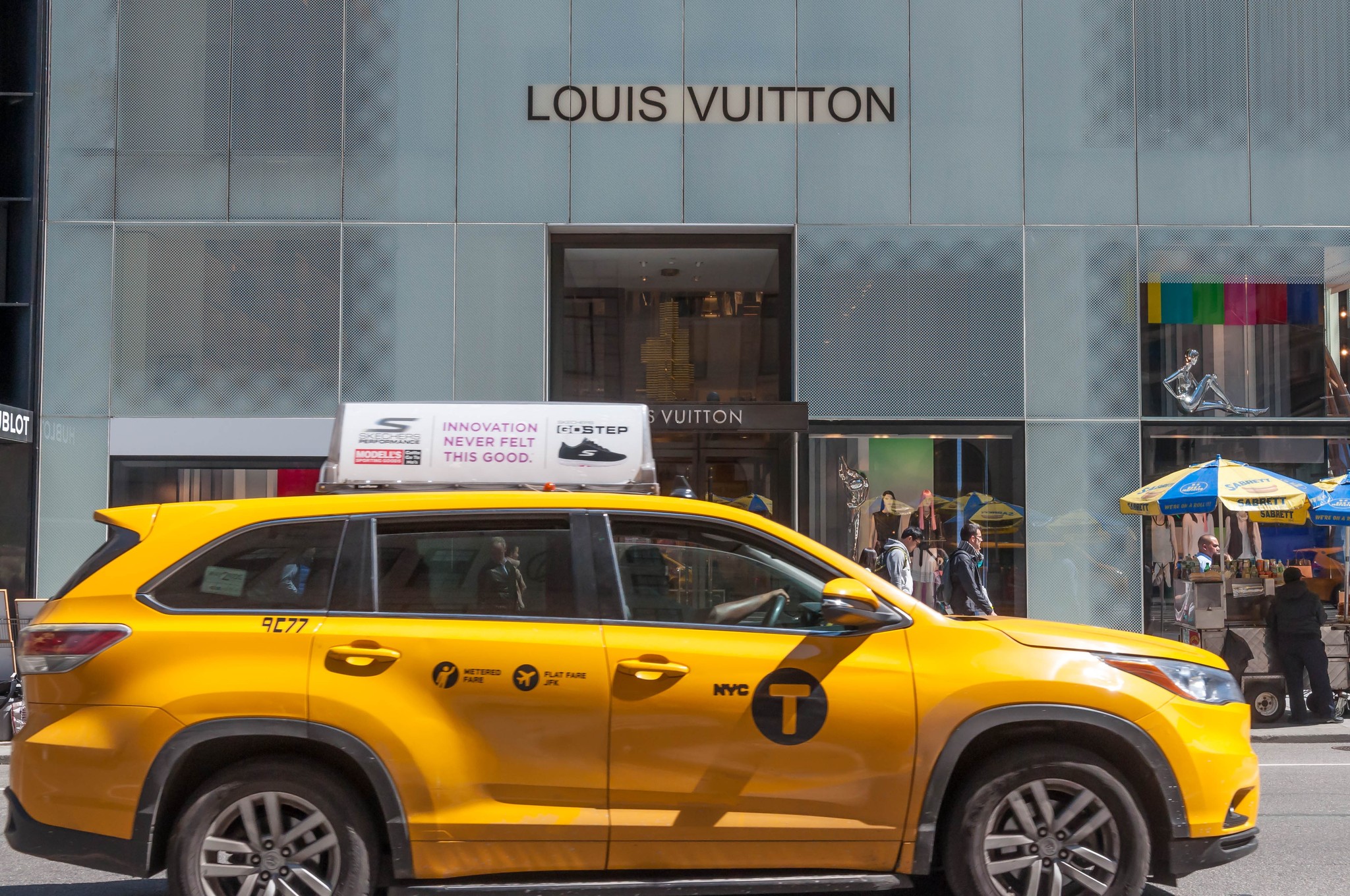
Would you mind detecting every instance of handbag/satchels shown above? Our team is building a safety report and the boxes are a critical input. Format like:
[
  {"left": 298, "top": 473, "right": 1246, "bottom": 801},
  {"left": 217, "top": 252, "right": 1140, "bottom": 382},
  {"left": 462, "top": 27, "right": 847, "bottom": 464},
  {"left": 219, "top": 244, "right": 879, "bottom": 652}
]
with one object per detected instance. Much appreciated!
[
  {"left": 935, "top": 564, "right": 943, "bottom": 589},
  {"left": 937, "top": 549, "right": 949, "bottom": 571}
]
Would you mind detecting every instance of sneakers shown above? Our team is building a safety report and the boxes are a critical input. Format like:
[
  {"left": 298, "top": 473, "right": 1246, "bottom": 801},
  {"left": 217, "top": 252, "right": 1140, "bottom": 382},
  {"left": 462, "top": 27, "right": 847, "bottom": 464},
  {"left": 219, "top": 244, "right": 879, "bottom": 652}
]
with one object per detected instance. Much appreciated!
[
  {"left": 1326, "top": 715, "right": 1344, "bottom": 723},
  {"left": 1287, "top": 716, "right": 1308, "bottom": 725}
]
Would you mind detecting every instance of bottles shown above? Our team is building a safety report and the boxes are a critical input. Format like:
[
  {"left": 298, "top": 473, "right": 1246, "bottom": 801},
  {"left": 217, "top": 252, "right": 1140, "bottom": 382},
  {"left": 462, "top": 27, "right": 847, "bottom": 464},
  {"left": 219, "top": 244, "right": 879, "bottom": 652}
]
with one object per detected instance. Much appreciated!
[
  {"left": 1242, "top": 559, "right": 1250, "bottom": 579},
  {"left": 1204, "top": 562, "right": 1210, "bottom": 573},
  {"left": 1276, "top": 559, "right": 1285, "bottom": 585},
  {"left": 1177, "top": 554, "right": 1200, "bottom": 581},
  {"left": 1250, "top": 560, "right": 1258, "bottom": 578},
  {"left": 1286, "top": 559, "right": 1289, "bottom": 566}
]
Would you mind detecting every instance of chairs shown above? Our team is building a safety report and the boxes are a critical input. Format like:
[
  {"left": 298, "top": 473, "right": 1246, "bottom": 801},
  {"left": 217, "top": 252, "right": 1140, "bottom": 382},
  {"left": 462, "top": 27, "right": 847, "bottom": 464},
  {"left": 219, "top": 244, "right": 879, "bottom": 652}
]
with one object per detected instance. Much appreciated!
[{"left": 618, "top": 549, "right": 686, "bottom": 619}]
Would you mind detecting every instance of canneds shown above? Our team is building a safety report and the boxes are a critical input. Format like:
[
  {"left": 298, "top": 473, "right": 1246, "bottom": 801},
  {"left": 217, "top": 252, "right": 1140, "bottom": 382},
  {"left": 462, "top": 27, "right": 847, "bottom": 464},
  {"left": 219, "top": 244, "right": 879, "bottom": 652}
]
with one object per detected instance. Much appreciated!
[
  {"left": 1224, "top": 559, "right": 1250, "bottom": 578},
  {"left": 1256, "top": 559, "right": 1277, "bottom": 584}
]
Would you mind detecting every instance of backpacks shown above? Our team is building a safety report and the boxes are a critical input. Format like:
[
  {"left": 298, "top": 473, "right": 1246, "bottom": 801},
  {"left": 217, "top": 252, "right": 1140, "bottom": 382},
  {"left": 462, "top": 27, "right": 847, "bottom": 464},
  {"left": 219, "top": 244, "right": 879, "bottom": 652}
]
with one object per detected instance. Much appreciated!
[
  {"left": 874, "top": 546, "right": 907, "bottom": 583},
  {"left": 936, "top": 550, "right": 972, "bottom": 606}
]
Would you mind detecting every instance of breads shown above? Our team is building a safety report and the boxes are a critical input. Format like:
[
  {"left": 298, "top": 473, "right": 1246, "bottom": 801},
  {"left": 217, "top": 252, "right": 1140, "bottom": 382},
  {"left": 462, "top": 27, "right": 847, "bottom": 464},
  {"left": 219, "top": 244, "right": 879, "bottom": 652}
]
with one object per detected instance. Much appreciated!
[
  {"left": 1337, "top": 602, "right": 1350, "bottom": 616},
  {"left": 1188, "top": 571, "right": 1222, "bottom": 581}
]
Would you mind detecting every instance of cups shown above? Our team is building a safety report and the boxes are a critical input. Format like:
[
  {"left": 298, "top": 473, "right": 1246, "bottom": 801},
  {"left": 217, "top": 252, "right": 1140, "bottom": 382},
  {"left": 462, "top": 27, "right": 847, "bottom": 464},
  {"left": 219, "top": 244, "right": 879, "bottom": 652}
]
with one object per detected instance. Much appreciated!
[{"left": 1339, "top": 592, "right": 1345, "bottom": 603}]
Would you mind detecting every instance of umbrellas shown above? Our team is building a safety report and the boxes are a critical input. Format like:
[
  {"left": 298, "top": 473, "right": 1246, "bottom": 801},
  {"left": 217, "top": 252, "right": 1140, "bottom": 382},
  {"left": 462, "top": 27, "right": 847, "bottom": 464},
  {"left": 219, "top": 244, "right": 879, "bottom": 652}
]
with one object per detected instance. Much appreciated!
[
  {"left": 700, "top": 490, "right": 777, "bottom": 520},
  {"left": 856, "top": 486, "right": 1026, "bottom": 538},
  {"left": 1117, "top": 448, "right": 1333, "bottom": 598},
  {"left": 1301, "top": 467, "right": 1350, "bottom": 624}
]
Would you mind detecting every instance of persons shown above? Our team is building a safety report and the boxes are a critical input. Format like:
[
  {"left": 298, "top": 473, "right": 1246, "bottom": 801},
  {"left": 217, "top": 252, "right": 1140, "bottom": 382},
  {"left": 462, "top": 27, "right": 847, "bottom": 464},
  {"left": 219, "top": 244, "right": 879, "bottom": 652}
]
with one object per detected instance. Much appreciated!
[
  {"left": 1189, "top": 534, "right": 1220, "bottom": 575},
  {"left": 907, "top": 490, "right": 944, "bottom": 612},
  {"left": 1267, "top": 567, "right": 1345, "bottom": 726},
  {"left": 1162, "top": 348, "right": 1271, "bottom": 417},
  {"left": 881, "top": 527, "right": 923, "bottom": 596},
  {"left": 868, "top": 488, "right": 904, "bottom": 583},
  {"left": 857, "top": 548, "right": 877, "bottom": 573},
  {"left": 941, "top": 521, "right": 997, "bottom": 619},
  {"left": 473, "top": 535, "right": 528, "bottom": 618},
  {"left": 620, "top": 548, "right": 792, "bottom": 635},
  {"left": 1147, "top": 502, "right": 1264, "bottom": 595}
]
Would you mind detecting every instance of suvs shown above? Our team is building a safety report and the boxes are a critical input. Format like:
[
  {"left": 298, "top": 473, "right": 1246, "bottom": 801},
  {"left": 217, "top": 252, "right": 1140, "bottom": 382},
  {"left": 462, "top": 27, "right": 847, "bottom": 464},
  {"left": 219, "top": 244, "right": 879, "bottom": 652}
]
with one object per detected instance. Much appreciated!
[{"left": 2, "top": 400, "right": 1263, "bottom": 896}]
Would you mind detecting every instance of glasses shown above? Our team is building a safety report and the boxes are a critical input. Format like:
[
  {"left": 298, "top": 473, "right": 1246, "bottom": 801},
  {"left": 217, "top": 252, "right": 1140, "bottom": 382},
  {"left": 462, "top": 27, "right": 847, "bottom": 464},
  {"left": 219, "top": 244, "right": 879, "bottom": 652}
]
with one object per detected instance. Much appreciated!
[
  {"left": 969, "top": 536, "right": 982, "bottom": 539},
  {"left": 914, "top": 540, "right": 920, "bottom": 547}
]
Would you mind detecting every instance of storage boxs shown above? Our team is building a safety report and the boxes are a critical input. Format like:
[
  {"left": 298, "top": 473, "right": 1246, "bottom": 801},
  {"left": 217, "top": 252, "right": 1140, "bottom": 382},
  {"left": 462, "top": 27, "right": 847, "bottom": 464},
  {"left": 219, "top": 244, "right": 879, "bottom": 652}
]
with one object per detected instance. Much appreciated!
[
  {"left": 1225, "top": 557, "right": 1256, "bottom": 572},
  {"left": 1337, "top": 590, "right": 1350, "bottom": 615}
]
